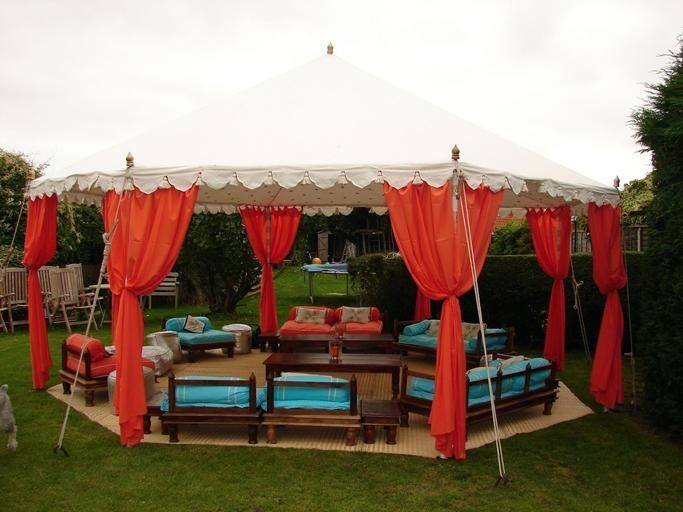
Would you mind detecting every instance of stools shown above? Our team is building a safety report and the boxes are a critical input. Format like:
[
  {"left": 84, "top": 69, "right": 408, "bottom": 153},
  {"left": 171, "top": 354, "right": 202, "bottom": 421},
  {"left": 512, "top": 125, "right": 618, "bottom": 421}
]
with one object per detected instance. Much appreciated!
[
  {"left": 141, "top": 344, "right": 174, "bottom": 383},
  {"left": 107, "top": 366, "right": 156, "bottom": 416},
  {"left": 221, "top": 324, "right": 253, "bottom": 354},
  {"left": 480, "top": 353, "right": 504, "bottom": 367},
  {"left": 145, "top": 331, "right": 182, "bottom": 364}
]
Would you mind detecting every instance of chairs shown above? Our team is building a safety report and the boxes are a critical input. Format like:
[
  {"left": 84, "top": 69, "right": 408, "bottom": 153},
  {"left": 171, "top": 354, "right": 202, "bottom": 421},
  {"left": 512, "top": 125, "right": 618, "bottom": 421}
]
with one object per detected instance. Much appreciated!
[
  {"left": 59, "top": 338, "right": 155, "bottom": 407},
  {"left": 0, "top": 263, "right": 180, "bottom": 334},
  {"left": 261, "top": 373, "right": 362, "bottom": 446},
  {"left": 161, "top": 319, "right": 237, "bottom": 362},
  {"left": 159, "top": 371, "right": 265, "bottom": 444}
]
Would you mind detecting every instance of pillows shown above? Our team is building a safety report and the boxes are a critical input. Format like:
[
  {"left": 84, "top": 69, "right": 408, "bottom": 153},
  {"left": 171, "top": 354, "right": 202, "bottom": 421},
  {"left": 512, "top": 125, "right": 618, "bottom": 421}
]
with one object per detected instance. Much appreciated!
[
  {"left": 66, "top": 333, "right": 105, "bottom": 361},
  {"left": 184, "top": 314, "right": 206, "bottom": 334},
  {"left": 411, "top": 355, "right": 552, "bottom": 400},
  {"left": 264, "top": 376, "right": 350, "bottom": 402},
  {"left": 341, "top": 306, "right": 371, "bottom": 324},
  {"left": 174, "top": 376, "right": 250, "bottom": 403},
  {"left": 294, "top": 307, "right": 326, "bottom": 324},
  {"left": 280, "top": 372, "right": 333, "bottom": 378},
  {"left": 403, "top": 319, "right": 508, "bottom": 349},
  {"left": 166, "top": 316, "right": 210, "bottom": 332}
]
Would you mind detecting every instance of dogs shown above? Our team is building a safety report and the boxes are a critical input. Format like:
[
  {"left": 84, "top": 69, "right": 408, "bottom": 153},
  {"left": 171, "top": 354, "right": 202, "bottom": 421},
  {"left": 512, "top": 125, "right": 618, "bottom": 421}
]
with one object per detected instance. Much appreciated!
[{"left": 0, "top": 382, "right": 18, "bottom": 452}]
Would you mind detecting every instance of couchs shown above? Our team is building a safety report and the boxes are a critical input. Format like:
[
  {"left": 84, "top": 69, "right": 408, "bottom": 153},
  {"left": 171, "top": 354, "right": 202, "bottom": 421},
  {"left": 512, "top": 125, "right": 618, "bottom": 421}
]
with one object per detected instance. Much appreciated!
[
  {"left": 398, "top": 354, "right": 559, "bottom": 441},
  {"left": 279, "top": 305, "right": 385, "bottom": 344},
  {"left": 394, "top": 316, "right": 515, "bottom": 368}
]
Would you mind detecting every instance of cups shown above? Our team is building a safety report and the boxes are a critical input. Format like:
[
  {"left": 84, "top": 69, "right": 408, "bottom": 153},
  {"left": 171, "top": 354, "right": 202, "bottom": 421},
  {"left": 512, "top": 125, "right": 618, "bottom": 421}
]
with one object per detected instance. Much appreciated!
[{"left": 329, "top": 341, "right": 343, "bottom": 361}]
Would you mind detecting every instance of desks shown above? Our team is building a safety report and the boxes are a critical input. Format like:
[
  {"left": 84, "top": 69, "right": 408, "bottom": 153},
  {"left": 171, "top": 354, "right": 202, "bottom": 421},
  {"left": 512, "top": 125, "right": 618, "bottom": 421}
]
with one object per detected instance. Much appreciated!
[{"left": 300, "top": 266, "right": 376, "bottom": 304}]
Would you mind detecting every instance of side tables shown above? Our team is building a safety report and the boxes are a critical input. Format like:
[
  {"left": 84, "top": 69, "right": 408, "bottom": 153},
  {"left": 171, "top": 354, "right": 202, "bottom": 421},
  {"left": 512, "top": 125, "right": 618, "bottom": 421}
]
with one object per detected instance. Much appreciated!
[
  {"left": 255, "top": 335, "right": 279, "bottom": 352},
  {"left": 361, "top": 400, "right": 401, "bottom": 444},
  {"left": 143, "top": 405, "right": 169, "bottom": 435}
]
